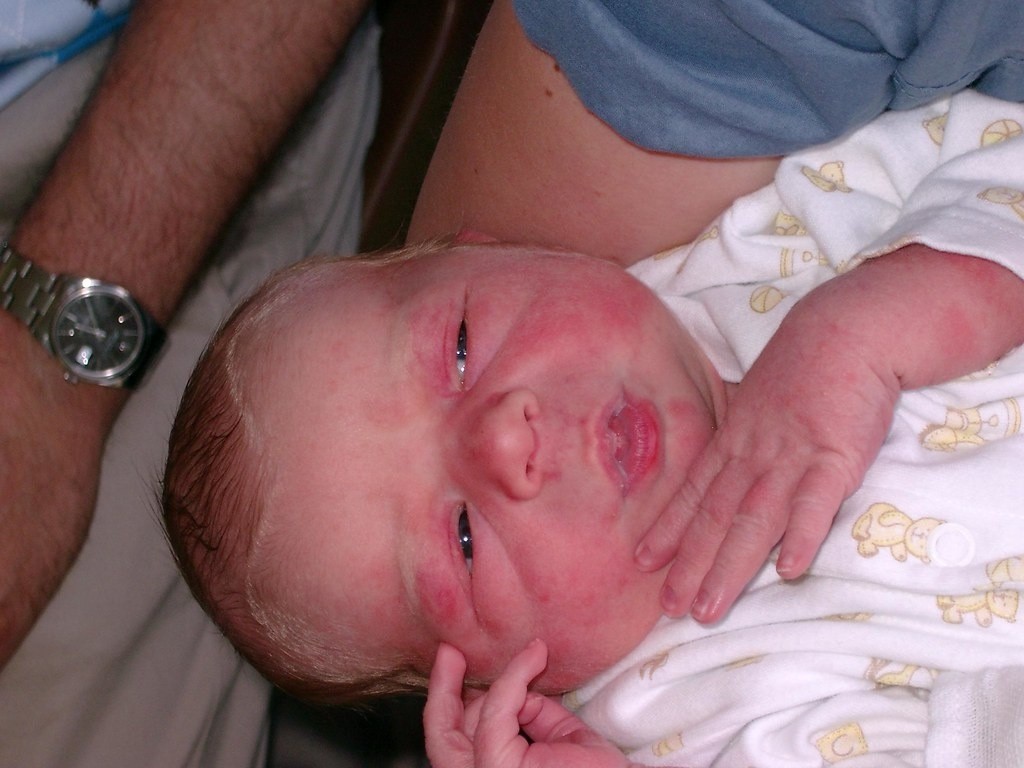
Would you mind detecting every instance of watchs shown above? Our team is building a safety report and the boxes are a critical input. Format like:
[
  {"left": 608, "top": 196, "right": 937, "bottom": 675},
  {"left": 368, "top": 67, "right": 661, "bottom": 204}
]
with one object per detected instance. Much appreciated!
[{"left": 0, "top": 235, "right": 171, "bottom": 396}]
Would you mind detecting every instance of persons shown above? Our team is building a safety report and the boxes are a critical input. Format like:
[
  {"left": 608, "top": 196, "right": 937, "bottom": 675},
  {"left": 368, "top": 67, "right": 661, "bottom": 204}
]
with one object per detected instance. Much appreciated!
[
  {"left": 155, "top": 89, "right": 1024, "bottom": 767},
  {"left": 0, "top": 1, "right": 1024, "bottom": 667}
]
